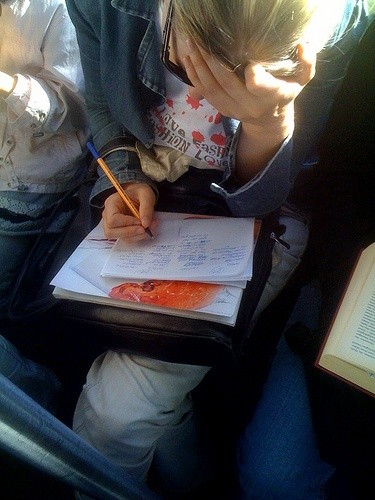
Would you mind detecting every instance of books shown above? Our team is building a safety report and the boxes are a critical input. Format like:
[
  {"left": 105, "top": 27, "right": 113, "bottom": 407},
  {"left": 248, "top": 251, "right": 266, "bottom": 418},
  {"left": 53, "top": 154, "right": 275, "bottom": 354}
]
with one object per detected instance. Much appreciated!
[
  {"left": 48, "top": 210, "right": 265, "bottom": 327},
  {"left": 312, "top": 240, "right": 375, "bottom": 395}
]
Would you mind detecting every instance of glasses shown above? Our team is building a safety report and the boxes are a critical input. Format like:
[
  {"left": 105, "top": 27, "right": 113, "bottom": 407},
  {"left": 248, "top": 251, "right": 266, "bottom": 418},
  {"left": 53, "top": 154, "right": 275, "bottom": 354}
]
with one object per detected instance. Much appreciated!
[{"left": 161, "top": 0, "right": 196, "bottom": 88}]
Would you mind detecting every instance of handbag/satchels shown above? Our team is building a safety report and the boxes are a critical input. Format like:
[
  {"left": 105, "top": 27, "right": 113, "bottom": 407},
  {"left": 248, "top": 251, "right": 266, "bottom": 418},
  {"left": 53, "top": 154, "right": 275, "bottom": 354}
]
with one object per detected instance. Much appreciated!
[{"left": 7, "top": 179, "right": 291, "bottom": 372}]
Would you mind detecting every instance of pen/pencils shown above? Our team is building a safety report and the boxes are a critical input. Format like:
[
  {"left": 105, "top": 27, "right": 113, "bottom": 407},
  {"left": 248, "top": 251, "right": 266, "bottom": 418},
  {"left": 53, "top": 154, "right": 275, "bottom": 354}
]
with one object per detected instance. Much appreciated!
[{"left": 86, "top": 141, "right": 155, "bottom": 241}]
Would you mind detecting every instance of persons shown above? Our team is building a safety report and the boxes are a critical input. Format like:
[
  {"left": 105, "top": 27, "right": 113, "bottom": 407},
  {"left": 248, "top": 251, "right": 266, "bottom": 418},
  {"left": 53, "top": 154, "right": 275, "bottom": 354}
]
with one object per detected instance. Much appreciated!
[
  {"left": 0, "top": 1, "right": 87, "bottom": 417},
  {"left": 238, "top": 20, "right": 375, "bottom": 500},
  {"left": 64, "top": 0, "right": 320, "bottom": 500}
]
